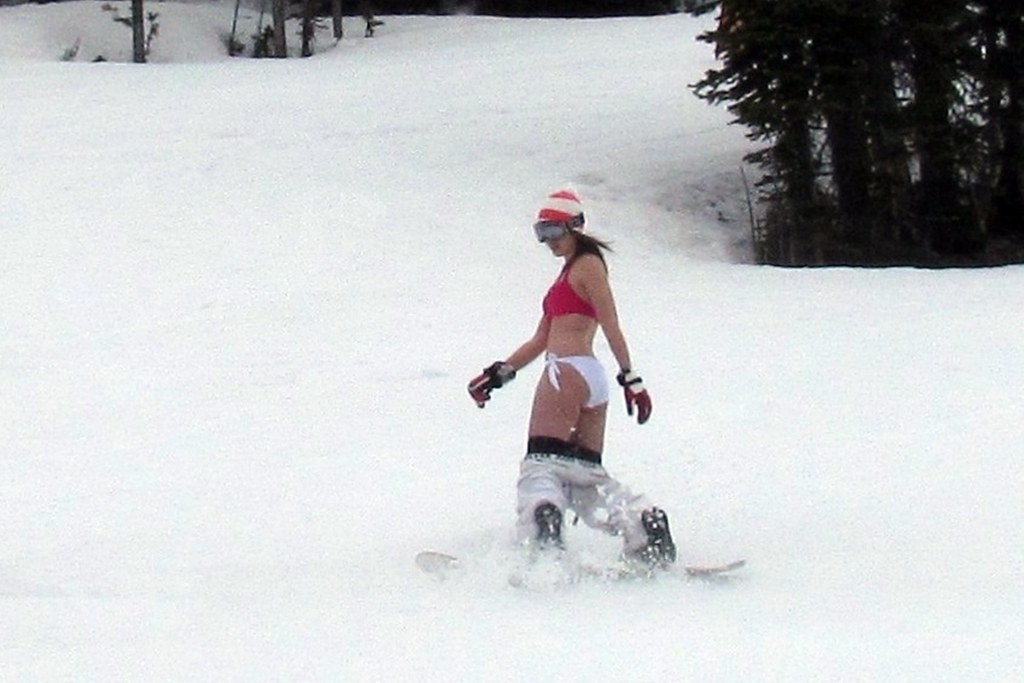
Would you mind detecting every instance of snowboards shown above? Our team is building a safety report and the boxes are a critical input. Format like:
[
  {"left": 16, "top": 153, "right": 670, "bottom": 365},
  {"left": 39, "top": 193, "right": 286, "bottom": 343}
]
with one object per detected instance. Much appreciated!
[{"left": 417, "top": 551, "right": 745, "bottom": 591}]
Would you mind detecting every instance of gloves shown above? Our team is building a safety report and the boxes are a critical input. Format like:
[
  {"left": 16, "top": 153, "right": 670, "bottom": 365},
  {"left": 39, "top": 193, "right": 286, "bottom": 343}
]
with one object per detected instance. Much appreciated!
[
  {"left": 466, "top": 362, "right": 516, "bottom": 406},
  {"left": 616, "top": 369, "right": 652, "bottom": 424}
]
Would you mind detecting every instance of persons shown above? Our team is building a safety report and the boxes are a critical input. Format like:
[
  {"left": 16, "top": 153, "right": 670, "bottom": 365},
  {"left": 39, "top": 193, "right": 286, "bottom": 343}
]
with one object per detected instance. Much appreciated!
[{"left": 469, "top": 191, "right": 677, "bottom": 569}]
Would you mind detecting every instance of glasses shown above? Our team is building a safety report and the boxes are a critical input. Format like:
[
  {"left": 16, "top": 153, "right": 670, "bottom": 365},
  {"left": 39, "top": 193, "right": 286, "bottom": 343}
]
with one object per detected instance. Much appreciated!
[{"left": 533, "top": 213, "right": 584, "bottom": 243}]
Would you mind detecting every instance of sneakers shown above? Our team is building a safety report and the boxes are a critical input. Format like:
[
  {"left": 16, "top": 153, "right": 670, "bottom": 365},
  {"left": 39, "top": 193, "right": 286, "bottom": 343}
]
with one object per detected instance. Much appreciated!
[
  {"left": 535, "top": 502, "right": 563, "bottom": 550},
  {"left": 642, "top": 505, "right": 676, "bottom": 563}
]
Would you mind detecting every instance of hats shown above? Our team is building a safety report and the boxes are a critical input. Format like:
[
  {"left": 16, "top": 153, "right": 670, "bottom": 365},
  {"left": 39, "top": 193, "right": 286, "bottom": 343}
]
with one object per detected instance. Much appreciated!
[{"left": 536, "top": 189, "right": 586, "bottom": 236}]
